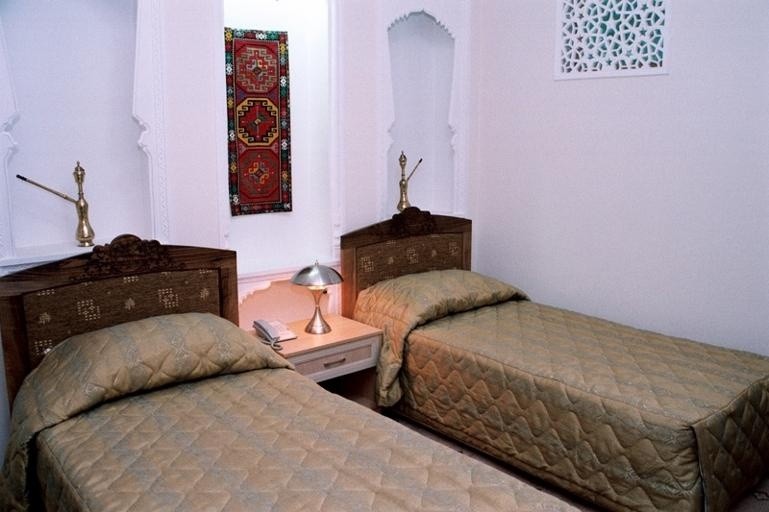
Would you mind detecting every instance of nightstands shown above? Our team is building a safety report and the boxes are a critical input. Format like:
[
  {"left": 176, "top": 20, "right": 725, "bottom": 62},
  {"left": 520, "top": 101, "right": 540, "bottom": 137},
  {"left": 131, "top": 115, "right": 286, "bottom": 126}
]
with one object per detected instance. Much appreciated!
[{"left": 245, "top": 313, "right": 382, "bottom": 385}]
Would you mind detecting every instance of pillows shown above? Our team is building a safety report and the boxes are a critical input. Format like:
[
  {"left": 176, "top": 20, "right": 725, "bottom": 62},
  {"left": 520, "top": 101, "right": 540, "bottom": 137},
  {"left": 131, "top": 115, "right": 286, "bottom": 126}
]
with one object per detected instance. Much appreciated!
[
  {"left": 359, "top": 269, "right": 518, "bottom": 333},
  {"left": 37, "top": 309, "right": 292, "bottom": 423}
]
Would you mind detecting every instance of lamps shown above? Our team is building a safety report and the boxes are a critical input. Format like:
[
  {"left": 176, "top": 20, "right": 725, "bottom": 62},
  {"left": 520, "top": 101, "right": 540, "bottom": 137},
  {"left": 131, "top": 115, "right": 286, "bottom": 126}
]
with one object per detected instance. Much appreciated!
[{"left": 291, "top": 263, "right": 344, "bottom": 337}]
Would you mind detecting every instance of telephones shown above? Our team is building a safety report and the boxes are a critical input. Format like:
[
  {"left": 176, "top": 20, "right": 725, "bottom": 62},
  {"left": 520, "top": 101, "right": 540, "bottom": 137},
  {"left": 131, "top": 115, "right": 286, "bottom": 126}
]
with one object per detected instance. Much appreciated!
[{"left": 253, "top": 319, "right": 296, "bottom": 342}]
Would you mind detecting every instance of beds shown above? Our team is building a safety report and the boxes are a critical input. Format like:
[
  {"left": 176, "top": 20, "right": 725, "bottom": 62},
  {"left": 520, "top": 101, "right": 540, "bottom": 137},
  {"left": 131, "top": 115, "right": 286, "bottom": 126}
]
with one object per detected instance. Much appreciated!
[
  {"left": 0, "top": 234, "right": 591, "bottom": 512},
  {"left": 341, "top": 208, "right": 769, "bottom": 512}
]
